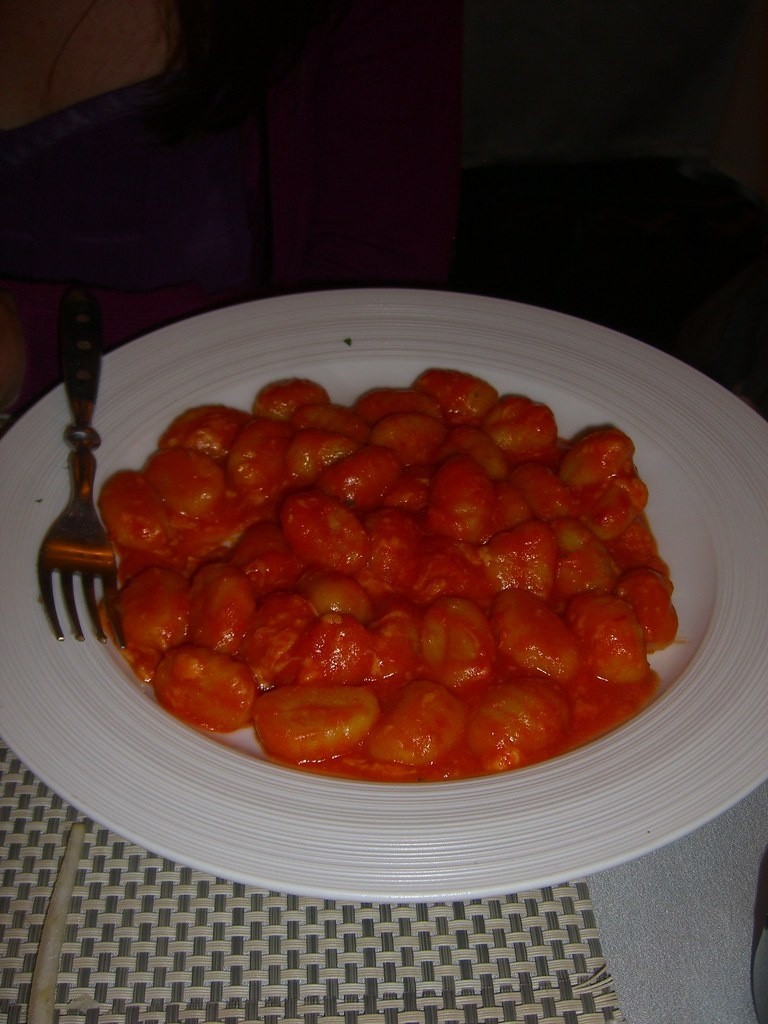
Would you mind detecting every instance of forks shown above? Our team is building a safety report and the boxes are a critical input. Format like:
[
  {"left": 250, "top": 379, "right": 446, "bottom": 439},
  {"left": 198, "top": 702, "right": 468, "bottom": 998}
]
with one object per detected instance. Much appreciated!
[{"left": 37, "top": 288, "right": 125, "bottom": 649}]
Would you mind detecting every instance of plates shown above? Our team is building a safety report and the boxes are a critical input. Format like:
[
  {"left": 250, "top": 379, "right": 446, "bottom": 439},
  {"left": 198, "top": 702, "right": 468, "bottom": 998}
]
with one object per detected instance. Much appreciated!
[{"left": 0, "top": 287, "right": 768, "bottom": 899}]
